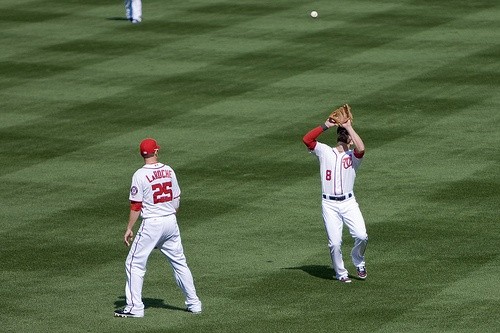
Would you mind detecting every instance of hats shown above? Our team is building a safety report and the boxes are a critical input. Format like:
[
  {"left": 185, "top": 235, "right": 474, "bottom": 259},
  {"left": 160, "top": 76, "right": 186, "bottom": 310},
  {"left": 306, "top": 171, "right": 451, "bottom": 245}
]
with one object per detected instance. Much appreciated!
[{"left": 140, "top": 138, "right": 160, "bottom": 157}]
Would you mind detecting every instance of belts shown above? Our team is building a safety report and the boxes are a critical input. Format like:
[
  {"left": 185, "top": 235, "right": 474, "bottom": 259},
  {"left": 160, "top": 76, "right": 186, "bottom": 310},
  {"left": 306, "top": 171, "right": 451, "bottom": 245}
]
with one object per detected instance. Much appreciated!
[{"left": 323, "top": 192, "right": 352, "bottom": 200}]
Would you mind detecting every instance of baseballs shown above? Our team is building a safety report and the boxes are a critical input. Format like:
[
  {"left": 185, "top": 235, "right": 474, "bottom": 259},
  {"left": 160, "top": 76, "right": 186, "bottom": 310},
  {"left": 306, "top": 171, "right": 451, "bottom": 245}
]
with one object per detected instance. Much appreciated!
[{"left": 310, "top": 10, "right": 319, "bottom": 18}]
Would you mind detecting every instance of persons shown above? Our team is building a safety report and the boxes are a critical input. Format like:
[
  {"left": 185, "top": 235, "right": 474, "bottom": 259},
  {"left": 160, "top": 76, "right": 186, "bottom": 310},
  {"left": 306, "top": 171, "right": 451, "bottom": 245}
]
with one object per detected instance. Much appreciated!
[
  {"left": 124, "top": 0, "right": 143, "bottom": 23},
  {"left": 302, "top": 102, "right": 368, "bottom": 283},
  {"left": 114, "top": 138, "right": 202, "bottom": 317}
]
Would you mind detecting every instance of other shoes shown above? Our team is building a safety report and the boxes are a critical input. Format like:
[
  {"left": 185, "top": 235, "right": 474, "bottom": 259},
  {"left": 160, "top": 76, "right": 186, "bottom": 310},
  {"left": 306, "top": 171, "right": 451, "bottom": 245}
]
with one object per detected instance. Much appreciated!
[
  {"left": 340, "top": 276, "right": 352, "bottom": 282},
  {"left": 132, "top": 19, "right": 138, "bottom": 23},
  {"left": 113, "top": 307, "right": 142, "bottom": 317},
  {"left": 356, "top": 266, "right": 367, "bottom": 278}
]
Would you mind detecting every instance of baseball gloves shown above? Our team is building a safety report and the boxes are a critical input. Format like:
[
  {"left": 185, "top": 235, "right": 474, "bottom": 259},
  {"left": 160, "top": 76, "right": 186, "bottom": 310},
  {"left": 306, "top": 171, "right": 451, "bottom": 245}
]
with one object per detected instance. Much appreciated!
[{"left": 328, "top": 103, "right": 354, "bottom": 125}]
